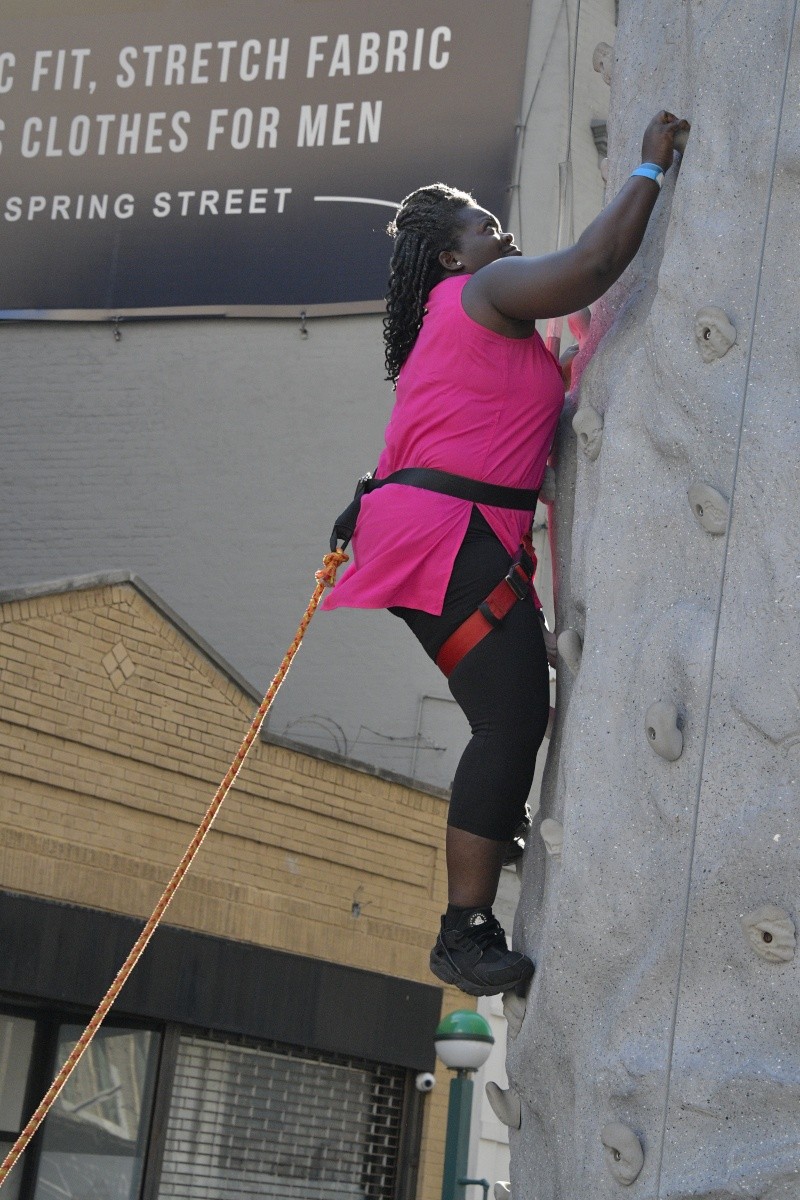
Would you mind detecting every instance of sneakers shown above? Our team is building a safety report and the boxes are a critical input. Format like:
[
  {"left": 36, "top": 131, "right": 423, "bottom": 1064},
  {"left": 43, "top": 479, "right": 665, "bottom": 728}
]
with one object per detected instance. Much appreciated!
[
  {"left": 429, "top": 905, "right": 536, "bottom": 997},
  {"left": 505, "top": 799, "right": 532, "bottom": 864}
]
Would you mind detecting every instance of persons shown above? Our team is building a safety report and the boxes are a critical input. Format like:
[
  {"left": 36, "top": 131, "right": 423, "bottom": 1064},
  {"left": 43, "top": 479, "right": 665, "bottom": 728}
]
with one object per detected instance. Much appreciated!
[{"left": 311, "top": 108, "right": 690, "bottom": 994}]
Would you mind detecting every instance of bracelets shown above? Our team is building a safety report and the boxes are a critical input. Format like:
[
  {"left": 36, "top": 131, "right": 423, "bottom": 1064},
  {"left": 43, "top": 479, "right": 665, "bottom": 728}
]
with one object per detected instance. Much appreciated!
[{"left": 628, "top": 163, "right": 665, "bottom": 191}]
